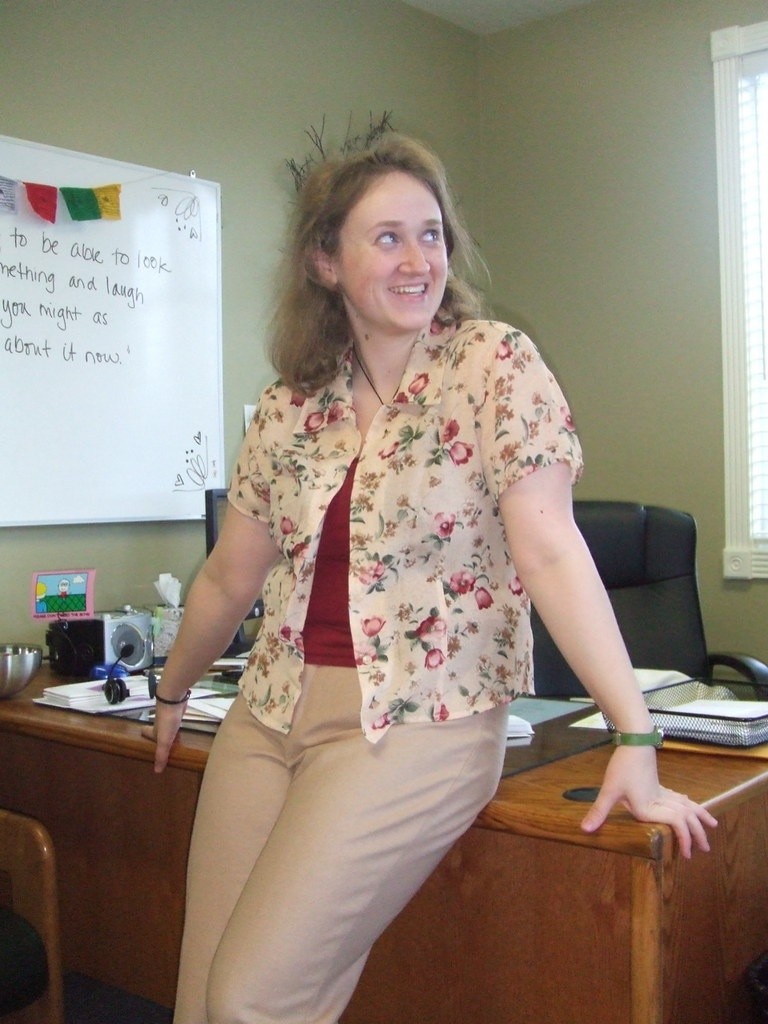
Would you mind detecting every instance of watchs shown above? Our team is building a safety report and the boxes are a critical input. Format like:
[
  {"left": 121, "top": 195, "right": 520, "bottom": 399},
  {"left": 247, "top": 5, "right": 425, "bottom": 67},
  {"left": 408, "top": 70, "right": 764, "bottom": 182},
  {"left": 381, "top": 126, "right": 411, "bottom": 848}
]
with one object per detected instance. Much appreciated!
[{"left": 612, "top": 725, "right": 664, "bottom": 749}]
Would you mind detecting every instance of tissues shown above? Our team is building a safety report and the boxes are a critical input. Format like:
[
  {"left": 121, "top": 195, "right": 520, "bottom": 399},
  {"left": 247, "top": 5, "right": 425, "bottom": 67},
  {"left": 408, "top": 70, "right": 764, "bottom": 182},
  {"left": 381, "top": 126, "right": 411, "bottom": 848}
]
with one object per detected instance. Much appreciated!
[{"left": 144, "top": 573, "right": 185, "bottom": 658}]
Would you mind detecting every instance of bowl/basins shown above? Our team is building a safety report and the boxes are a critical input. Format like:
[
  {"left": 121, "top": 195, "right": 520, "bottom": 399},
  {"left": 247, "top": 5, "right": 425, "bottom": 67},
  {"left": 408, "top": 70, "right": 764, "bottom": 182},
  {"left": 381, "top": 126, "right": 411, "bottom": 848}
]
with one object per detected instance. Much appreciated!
[{"left": 0, "top": 643, "right": 42, "bottom": 700}]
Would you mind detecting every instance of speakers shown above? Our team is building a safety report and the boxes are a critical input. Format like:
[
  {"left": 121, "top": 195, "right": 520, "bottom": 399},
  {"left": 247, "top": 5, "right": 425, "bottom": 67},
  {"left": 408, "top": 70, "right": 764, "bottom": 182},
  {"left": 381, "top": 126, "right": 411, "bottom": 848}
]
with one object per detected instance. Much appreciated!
[{"left": 46, "top": 608, "right": 155, "bottom": 676}]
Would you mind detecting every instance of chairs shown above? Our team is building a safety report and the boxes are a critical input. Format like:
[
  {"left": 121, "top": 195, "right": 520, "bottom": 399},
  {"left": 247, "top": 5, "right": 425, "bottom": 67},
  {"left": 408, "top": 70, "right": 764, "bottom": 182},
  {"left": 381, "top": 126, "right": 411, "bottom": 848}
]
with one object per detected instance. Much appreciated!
[{"left": 521, "top": 500, "right": 768, "bottom": 699}]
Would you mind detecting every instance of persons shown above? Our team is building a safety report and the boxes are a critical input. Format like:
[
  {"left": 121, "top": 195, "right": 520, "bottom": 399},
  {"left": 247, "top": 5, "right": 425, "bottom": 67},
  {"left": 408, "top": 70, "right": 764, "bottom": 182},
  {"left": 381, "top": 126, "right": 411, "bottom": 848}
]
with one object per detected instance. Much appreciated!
[{"left": 139, "top": 130, "right": 719, "bottom": 1024}]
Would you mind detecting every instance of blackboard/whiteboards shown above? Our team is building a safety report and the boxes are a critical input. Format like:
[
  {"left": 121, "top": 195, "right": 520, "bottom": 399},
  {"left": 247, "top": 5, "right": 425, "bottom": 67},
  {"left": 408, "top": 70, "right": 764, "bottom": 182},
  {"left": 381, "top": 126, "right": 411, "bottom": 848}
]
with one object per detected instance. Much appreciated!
[{"left": 0, "top": 134, "right": 226, "bottom": 527}]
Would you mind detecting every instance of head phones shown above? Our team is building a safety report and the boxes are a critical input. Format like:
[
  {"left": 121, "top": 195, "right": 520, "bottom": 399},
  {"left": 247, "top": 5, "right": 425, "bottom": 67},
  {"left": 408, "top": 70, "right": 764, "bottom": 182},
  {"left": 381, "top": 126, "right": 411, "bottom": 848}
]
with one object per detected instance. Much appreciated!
[{"left": 102, "top": 671, "right": 159, "bottom": 704}]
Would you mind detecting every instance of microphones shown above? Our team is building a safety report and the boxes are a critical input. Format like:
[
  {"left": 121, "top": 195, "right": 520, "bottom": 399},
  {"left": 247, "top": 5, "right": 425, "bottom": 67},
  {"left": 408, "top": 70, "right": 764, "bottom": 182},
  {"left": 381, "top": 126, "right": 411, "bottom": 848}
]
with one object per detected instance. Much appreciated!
[{"left": 108, "top": 643, "right": 134, "bottom": 681}]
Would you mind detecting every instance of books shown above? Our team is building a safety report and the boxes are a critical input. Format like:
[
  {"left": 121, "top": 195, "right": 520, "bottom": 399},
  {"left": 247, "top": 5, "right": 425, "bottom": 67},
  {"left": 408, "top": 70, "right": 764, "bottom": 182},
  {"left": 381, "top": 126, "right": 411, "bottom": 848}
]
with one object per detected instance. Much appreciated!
[{"left": 43, "top": 674, "right": 238, "bottom": 733}]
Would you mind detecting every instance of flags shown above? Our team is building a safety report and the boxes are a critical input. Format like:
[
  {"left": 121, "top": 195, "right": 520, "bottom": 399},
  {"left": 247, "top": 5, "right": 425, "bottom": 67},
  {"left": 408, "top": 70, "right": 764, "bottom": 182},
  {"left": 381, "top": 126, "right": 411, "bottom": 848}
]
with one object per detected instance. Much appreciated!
[{"left": 0, "top": 176, "right": 124, "bottom": 223}]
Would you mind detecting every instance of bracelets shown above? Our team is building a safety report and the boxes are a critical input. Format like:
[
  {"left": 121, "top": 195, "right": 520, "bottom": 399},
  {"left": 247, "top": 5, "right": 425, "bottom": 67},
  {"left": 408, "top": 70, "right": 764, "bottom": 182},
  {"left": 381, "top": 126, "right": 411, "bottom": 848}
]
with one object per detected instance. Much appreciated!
[{"left": 154, "top": 683, "right": 191, "bottom": 705}]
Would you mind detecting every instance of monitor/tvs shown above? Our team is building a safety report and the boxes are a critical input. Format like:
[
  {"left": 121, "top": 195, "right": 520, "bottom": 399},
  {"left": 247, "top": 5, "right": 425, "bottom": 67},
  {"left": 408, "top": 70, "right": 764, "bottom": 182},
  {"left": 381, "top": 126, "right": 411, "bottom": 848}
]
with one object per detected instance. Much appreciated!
[{"left": 204, "top": 487, "right": 265, "bottom": 656}]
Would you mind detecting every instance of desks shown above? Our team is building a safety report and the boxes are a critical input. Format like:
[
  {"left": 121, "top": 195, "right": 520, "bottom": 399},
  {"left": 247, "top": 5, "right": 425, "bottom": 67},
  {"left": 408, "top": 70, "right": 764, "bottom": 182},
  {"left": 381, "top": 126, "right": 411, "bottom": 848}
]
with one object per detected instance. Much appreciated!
[{"left": 1, "top": 663, "right": 768, "bottom": 1024}]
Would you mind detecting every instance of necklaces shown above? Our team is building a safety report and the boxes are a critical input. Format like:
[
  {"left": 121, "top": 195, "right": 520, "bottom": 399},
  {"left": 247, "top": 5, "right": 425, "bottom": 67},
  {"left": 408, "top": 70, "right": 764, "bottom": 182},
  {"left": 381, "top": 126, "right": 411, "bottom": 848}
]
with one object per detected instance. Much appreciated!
[{"left": 352, "top": 342, "right": 384, "bottom": 406}]
